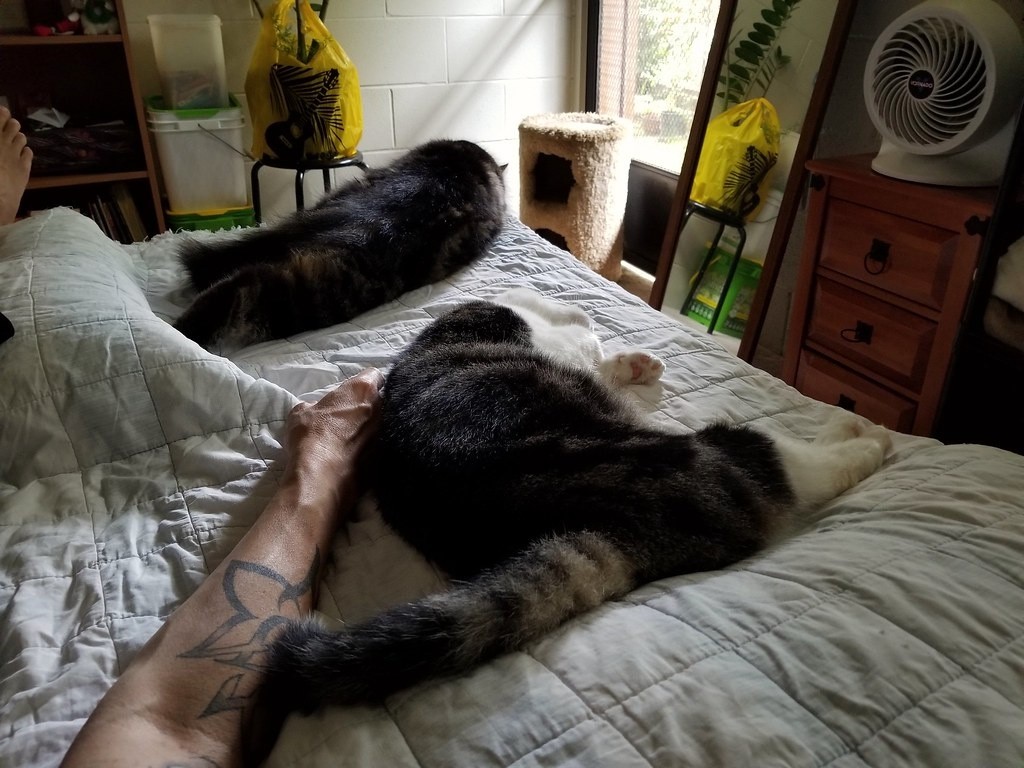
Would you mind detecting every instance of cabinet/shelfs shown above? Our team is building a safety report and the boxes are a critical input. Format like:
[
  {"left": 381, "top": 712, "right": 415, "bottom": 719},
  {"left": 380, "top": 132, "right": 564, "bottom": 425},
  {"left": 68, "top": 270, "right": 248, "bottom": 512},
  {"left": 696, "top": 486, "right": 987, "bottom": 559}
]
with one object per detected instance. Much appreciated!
[
  {"left": 0, "top": 0, "right": 166, "bottom": 242},
  {"left": 783, "top": 154, "right": 1003, "bottom": 438}
]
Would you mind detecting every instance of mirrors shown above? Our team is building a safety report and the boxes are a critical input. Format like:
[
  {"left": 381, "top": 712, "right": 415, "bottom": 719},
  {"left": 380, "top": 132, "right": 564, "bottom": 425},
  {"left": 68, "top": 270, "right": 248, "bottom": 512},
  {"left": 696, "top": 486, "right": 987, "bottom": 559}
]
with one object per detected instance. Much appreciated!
[{"left": 649, "top": 1, "right": 859, "bottom": 364}]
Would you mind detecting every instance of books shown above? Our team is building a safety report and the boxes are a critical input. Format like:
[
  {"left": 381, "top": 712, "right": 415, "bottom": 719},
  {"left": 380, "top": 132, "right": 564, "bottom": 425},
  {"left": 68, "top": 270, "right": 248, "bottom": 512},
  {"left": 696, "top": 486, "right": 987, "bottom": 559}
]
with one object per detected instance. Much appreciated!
[{"left": 86, "top": 190, "right": 151, "bottom": 245}]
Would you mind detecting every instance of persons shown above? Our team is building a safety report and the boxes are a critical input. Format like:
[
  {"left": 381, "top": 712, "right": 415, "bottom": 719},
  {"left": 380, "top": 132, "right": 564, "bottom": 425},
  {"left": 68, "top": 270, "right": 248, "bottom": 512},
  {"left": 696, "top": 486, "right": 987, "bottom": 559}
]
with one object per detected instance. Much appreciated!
[{"left": 0, "top": 104, "right": 388, "bottom": 768}]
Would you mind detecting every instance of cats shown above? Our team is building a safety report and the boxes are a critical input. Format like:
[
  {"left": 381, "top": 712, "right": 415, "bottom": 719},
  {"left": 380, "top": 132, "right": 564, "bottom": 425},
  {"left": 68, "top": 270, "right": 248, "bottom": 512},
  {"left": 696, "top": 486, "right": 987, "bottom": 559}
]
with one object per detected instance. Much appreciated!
[
  {"left": 169, "top": 136, "right": 509, "bottom": 352},
  {"left": 233, "top": 286, "right": 894, "bottom": 768}
]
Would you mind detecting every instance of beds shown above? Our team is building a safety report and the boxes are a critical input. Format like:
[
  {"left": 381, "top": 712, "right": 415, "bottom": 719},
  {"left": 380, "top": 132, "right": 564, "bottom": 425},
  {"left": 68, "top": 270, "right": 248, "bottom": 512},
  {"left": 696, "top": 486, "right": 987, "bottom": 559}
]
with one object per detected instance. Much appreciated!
[{"left": 0, "top": 189, "right": 1024, "bottom": 767}]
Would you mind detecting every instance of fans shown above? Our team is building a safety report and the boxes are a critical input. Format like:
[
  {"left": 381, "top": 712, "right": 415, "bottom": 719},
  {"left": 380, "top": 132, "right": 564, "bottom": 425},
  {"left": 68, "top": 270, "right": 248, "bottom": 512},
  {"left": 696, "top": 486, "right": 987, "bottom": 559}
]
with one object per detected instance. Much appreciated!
[{"left": 864, "top": 0, "right": 1023, "bottom": 189}]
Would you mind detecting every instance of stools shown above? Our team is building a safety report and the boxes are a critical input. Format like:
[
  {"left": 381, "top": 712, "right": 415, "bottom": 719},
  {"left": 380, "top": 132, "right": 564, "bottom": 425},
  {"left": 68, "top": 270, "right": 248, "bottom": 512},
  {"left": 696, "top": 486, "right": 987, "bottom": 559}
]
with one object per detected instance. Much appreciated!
[
  {"left": 249, "top": 149, "right": 370, "bottom": 228},
  {"left": 682, "top": 199, "right": 746, "bottom": 339}
]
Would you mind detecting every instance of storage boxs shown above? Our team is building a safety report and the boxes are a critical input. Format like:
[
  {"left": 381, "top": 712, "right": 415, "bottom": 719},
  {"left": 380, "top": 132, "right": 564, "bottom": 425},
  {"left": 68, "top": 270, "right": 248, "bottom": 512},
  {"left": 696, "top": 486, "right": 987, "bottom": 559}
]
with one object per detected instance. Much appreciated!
[
  {"left": 684, "top": 126, "right": 802, "bottom": 339},
  {"left": 140, "top": 10, "right": 257, "bottom": 232}
]
